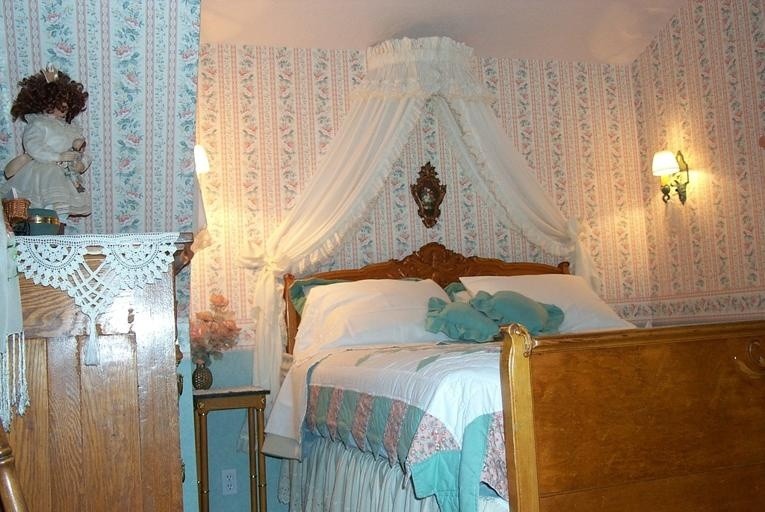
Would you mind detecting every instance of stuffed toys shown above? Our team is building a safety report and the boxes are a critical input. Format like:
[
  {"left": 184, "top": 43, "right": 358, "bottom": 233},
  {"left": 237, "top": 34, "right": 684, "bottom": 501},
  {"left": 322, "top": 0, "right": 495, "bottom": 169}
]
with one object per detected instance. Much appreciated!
[
  {"left": 3, "top": 60, "right": 93, "bottom": 235},
  {"left": 57, "top": 138, "right": 87, "bottom": 193}
]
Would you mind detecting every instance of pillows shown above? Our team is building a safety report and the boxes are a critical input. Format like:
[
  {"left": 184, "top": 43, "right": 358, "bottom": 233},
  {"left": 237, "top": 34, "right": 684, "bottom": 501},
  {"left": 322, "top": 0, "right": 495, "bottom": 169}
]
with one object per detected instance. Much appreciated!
[
  {"left": 467, "top": 290, "right": 564, "bottom": 335},
  {"left": 293, "top": 279, "right": 459, "bottom": 360},
  {"left": 443, "top": 281, "right": 463, "bottom": 301},
  {"left": 458, "top": 273, "right": 638, "bottom": 333},
  {"left": 425, "top": 297, "right": 502, "bottom": 343},
  {"left": 287, "top": 277, "right": 422, "bottom": 316}
]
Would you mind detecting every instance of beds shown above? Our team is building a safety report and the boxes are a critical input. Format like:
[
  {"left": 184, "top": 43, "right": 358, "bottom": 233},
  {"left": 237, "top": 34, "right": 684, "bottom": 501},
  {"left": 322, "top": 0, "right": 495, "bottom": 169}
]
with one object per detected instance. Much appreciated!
[{"left": 260, "top": 237, "right": 765, "bottom": 511}]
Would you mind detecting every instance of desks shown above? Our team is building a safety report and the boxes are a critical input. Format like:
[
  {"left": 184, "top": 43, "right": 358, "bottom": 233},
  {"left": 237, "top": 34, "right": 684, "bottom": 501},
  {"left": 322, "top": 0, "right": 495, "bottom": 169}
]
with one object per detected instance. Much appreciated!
[{"left": 190, "top": 383, "right": 275, "bottom": 511}]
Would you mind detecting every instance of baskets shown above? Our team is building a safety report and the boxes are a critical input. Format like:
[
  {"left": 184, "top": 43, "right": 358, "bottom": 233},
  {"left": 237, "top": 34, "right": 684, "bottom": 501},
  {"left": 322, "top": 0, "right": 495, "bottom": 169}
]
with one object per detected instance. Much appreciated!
[{"left": 0, "top": 199, "right": 32, "bottom": 222}]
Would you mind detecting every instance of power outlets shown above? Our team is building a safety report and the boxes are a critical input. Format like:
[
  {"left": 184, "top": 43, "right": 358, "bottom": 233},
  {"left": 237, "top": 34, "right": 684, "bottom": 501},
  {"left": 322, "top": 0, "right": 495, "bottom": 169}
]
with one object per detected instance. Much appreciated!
[{"left": 220, "top": 467, "right": 238, "bottom": 496}]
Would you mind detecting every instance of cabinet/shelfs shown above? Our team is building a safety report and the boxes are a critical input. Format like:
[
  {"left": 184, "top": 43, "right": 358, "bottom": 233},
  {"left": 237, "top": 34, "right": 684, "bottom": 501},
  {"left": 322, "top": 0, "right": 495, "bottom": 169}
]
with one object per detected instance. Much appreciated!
[{"left": 0, "top": 229, "right": 200, "bottom": 512}]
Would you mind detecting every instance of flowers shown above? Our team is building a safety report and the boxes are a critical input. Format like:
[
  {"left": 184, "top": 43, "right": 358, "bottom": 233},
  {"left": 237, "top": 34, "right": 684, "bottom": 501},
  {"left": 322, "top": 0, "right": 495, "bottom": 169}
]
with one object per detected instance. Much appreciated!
[{"left": 185, "top": 289, "right": 243, "bottom": 371}]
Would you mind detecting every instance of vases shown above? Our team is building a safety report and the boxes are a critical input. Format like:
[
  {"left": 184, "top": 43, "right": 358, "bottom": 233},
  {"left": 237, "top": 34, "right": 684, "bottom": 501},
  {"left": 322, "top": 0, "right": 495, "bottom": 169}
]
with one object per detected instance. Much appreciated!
[{"left": 192, "top": 355, "right": 213, "bottom": 391}]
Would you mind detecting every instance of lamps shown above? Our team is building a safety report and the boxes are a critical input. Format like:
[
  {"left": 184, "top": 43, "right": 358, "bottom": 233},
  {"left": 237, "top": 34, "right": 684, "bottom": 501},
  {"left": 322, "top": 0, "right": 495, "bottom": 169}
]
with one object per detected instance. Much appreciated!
[{"left": 648, "top": 148, "right": 692, "bottom": 206}]
